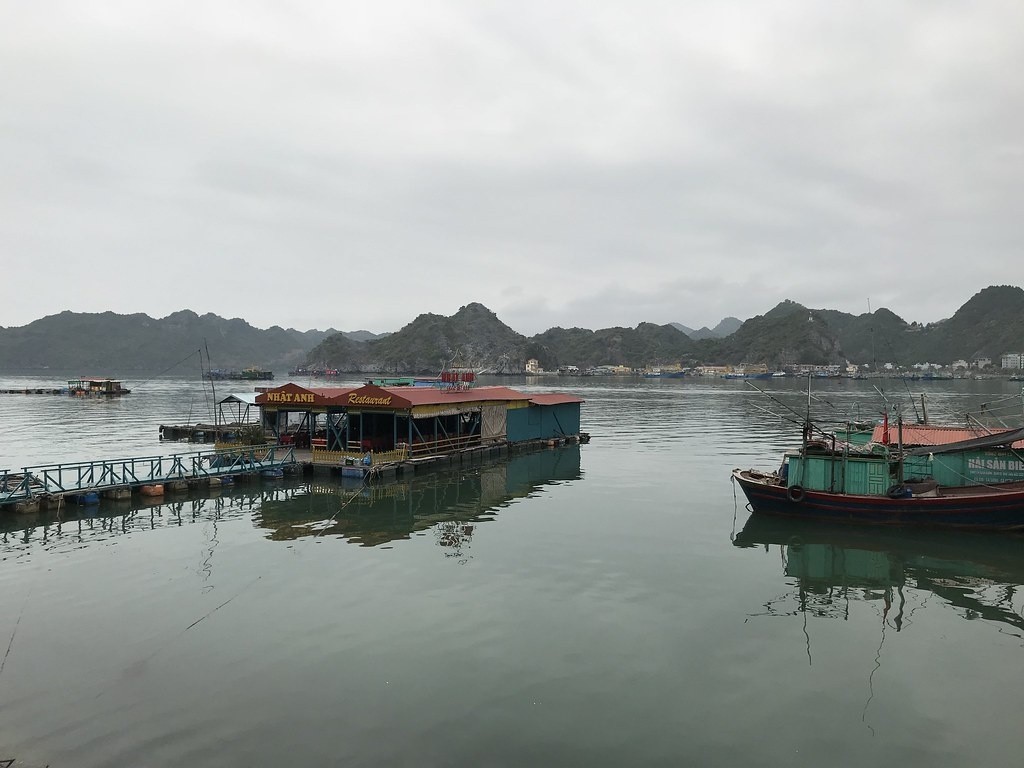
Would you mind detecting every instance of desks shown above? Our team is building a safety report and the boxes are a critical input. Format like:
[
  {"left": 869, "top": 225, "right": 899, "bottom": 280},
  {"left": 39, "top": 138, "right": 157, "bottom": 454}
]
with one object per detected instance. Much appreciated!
[
  {"left": 282, "top": 435, "right": 296, "bottom": 449},
  {"left": 362, "top": 439, "right": 382, "bottom": 451},
  {"left": 312, "top": 441, "right": 326, "bottom": 451}
]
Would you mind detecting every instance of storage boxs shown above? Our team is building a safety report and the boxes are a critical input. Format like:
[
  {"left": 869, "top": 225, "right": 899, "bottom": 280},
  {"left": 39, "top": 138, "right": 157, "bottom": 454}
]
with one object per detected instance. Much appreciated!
[
  {"left": 786, "top": 455, "right": 842, "bottom": 495},
  {"left": 844, "top": 458, "right": 889, "bottom": 497}
]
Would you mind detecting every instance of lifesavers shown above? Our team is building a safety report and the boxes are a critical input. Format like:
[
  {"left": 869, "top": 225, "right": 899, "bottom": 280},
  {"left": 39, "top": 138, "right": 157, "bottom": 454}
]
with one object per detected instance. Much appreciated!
[{"left": 787, "top": 485, "right": 805, "bottom": 504}]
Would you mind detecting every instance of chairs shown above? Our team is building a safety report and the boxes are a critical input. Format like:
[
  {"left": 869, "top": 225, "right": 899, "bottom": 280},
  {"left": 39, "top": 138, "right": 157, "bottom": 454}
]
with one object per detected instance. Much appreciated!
[{"left": 280, "top": 429, "right": 469, "bottom": 454}]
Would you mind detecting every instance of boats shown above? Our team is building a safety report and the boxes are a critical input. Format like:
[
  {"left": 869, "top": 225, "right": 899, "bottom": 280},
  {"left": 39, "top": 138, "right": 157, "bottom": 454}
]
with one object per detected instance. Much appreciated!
[
  {"left": 288, "top": 363, "right": 340, "bottom": 376},
  {"left": 202, "top": 366, "right": 275, "bottom": 380},
  {"left": 729, "top": 295, "right": 1024, "bottom": 527}
]
